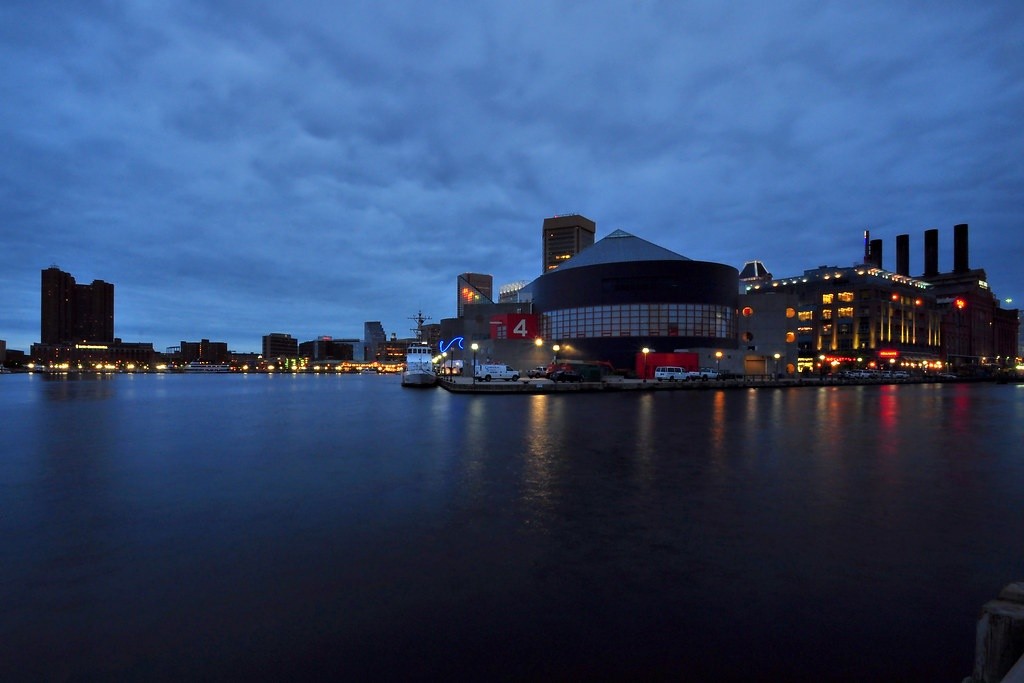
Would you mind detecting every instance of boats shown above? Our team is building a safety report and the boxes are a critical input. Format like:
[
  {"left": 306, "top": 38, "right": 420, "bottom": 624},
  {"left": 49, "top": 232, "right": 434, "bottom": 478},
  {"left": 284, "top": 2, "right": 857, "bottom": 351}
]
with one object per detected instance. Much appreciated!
[{"left": 401, "top": 310, "right": 437, "bottom": 386}]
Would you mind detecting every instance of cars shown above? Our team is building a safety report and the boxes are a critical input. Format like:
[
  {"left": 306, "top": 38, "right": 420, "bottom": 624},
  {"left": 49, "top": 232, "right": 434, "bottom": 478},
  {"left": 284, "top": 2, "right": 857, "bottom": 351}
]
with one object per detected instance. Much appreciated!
[{"left": 839, "top": 370, "right": 910, "bottom": 379}]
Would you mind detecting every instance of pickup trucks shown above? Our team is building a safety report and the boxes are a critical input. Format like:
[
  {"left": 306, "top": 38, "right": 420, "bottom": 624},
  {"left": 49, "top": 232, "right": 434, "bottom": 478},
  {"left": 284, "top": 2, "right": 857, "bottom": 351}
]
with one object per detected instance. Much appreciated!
[{"left": 689, "top": 367, "right": 722, "bottom": 382}]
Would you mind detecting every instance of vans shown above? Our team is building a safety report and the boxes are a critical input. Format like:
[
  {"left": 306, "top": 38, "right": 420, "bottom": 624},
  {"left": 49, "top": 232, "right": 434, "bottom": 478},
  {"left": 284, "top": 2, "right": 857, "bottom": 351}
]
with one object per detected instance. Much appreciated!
[{"left": 655, "top": 366, "right": 690, "bottom": 382}]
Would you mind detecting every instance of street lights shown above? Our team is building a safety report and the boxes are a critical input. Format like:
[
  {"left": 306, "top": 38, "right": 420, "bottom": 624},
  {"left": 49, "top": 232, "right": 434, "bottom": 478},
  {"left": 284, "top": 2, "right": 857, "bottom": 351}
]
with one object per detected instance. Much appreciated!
[
  {"left": 774, "top": 354, "right": 780, "bottom": 381},
  {"left": 553, "top": 345, "right": 560, "bottom": 382},
  {"left": 923, "top": 361, "right": 928, "bottom": 371},
  {"left": 438, "top": 352, "right": 447, "bottom": 380},
  {"left": 716, "top": 351, "right": 722, "bottom": 382},
  {"left": 642, "top": 347, "right": 649, "bottom": 383},
  {"left": 450, "top": 347, "right": 454, "bottom": 382},
  {"left": 471, "top": 343, "right": 478, "bottom": 385},
  {"left": 857, "top": 358, "right": 863, "bottom": 380}
]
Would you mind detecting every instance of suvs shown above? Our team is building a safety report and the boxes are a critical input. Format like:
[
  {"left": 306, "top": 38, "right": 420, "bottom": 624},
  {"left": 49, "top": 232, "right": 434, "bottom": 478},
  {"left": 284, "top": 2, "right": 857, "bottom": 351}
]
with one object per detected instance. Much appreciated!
[{"left": 526, "top": 365, "right": 585, "bottom": 383}]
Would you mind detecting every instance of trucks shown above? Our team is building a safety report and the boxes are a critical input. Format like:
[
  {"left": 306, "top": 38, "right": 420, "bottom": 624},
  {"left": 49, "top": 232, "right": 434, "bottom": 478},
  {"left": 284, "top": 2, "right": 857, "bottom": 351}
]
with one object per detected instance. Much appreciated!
[{"left": 475, "top": 365, "right": 520, "bottom": 382}]
